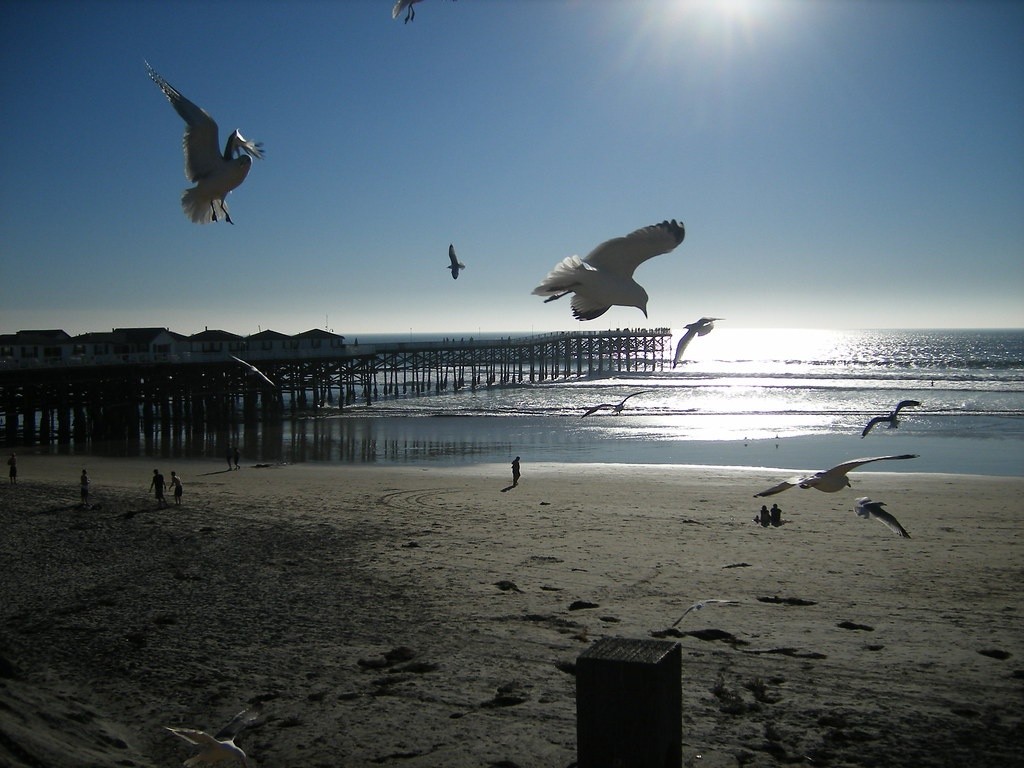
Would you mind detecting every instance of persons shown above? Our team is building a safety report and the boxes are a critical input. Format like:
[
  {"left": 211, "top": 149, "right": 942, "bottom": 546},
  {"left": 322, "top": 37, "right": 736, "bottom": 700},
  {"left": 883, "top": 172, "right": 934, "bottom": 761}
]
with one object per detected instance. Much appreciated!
[
  {"left": 761, "top": 505, "right": 769, "bottom": 521},
  {"left": 169, "top": 471, "right": 182, "bottom": 504},
  {"left": 148, "top": 469, "right": 167, "bottom": 503},
  {"left": 234, "top": 448, "right": 240, "bottom": 469},
  {"left": 770, "top": 504, "right": 782, "bottom": 521},
  {"left": 511, "top": 456, "right": 520, "bottom": 486},
  {"left": 7, "top": 453, "right": 17, "bottom": 484},
  {"left": 224, "top": 444, "right": 233, "bottom": 471},
  {"left": 81, "top": 468, "right": 91, "bottom": 505}
]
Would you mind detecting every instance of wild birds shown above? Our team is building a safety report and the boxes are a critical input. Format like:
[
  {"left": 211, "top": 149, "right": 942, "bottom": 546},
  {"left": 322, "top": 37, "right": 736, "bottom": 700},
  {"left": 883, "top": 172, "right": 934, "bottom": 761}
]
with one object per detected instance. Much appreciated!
[
  {"left": 859, "top": 400, "right": 923, "bottom": 439},
  {"left": 161, "top": 699, "right": 268, "bottom": 768},
  {"left": 391, "top": 0, "right": 425, "bottom": 25},
  {"left": 850, "top": 496, "right": 911, "bottom": 539},
  {"left": 446, "top": 242, "right": 466, "bottom": 280},
  {"left": 228, "top": 351, "right": 275, "bottom": 388},
  {"left": 529, "top": 219, "right": 685, "bottom": 322},
  {"left": 672, "top": 317, "right": 726, "bottom": 369},
  {"left": 142, "top": 55, "right": 266, "bottom": 224},
  {"left": 579, "top": 389, "right": 660, "bottom": 420},
  {"left": 751, "top": 453, "right": 921, "bottom": 498}
]
